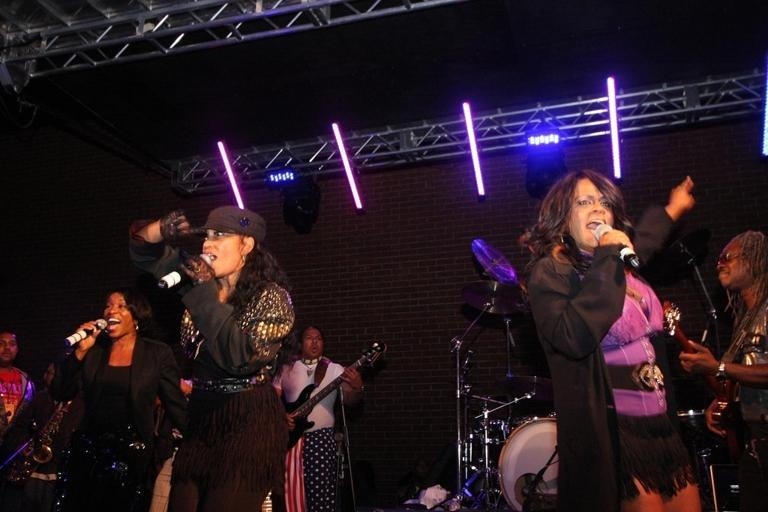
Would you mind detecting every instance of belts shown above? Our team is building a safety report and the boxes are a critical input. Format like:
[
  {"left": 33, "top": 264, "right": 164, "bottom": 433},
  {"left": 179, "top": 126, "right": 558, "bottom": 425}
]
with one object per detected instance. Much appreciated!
[
  {"left": 605, "top": 362, "right": 664, "bottom": 391},
  {"left": 190, "top": 373, "right": 254, "bottom": 393}
]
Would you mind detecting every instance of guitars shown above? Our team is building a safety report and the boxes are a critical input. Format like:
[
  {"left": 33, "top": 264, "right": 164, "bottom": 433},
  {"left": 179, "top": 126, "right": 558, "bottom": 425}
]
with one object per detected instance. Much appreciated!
[
  {"left": 660, "top": 300, "right": 749, "bottom": 466},
  {"left": 283, "top": 339, "right": 388, "bottom": 447}
]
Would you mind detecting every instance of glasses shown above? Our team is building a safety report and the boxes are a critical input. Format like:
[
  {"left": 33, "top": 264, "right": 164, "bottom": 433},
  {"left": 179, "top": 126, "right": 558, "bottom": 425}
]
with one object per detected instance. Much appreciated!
[{"left": 717, "top": 252, "right": 742, "bottom": 262}]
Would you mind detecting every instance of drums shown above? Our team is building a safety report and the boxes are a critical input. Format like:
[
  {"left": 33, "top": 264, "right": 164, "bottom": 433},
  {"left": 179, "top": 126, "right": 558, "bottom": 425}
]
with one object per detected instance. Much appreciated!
[{"left": 490, "top": 416, "right": 559, "bottom": 512}]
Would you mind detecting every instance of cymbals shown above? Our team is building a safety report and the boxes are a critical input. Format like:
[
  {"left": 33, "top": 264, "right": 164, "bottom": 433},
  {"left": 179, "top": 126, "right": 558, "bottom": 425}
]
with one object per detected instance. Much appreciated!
[
  {"left": 497, "top": 375, "right": 554, "bottom": 401},
  {"left": 472, "top": 238, "right": 517, "bottom": 280},
  {"left": 462, "top": 280, "right": 525, "bottom": 314}
]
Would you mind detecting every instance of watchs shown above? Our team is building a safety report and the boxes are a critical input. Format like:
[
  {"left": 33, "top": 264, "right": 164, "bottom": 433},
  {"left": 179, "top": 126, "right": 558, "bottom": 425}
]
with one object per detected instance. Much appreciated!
[{"left": 714, "top": 360, "right": 727, "bottom": 384}]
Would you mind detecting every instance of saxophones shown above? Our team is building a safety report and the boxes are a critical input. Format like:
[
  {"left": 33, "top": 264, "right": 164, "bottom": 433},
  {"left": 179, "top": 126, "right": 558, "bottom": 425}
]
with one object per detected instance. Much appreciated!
[{"left": 6, "top": 401, "right": 73, "bottom": 485}]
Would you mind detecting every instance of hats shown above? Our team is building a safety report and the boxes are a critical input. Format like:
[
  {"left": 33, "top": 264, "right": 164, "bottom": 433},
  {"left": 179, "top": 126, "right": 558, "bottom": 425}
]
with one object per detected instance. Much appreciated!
[{"left": 206, "top": 205, "right": 265, "bottom": 241}]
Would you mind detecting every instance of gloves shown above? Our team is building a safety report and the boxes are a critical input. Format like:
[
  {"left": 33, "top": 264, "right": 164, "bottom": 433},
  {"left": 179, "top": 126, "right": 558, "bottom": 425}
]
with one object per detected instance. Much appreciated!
[
  {"left": 160, "top": 211, "right": 204, "bottom": 241},
  {"left": 177, "top": 256, "right": 213, "bottom": 286}
]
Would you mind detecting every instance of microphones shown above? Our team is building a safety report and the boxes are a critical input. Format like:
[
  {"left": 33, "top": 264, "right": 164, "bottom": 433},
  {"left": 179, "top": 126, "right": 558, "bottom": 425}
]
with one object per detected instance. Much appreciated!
[
  {"left": 65, "top": 318, "right": 108, "bottom": 347},
  {"left": 594, "top": 224, "right": 640, "bottom": 268},
  {"left": 158, "top": 253, "right": 212, "bottom": 289}
]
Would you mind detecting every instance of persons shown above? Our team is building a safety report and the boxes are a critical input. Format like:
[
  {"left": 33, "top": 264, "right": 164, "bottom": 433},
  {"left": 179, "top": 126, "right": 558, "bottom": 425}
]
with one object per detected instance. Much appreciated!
[
  {"left": 48, "top": 287, "right": 188, "bottom": 512},
  {"left": 127, "top": 204, "right": 296, "bottom": 512},
  {"left": 0, "top": 329, "right": 35, "bottom": 426},
  {"left": 273, "top": 326, "right": 365, "bottom": 512},
  {"left": 680, "top": 231, "right": 768, "bottom": 472},
  {"left": 519, "top": 169, "right": 701, "bottom": 512},
  {"left": 18, "top": 361, "right": 84, "bottom": 511}
]
